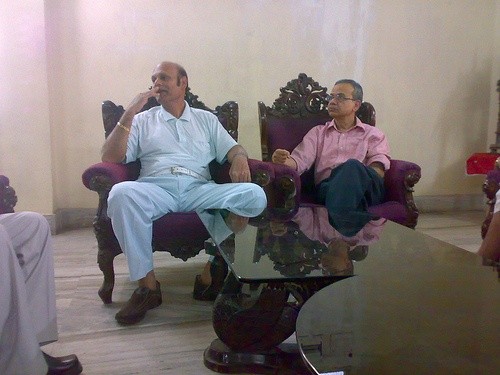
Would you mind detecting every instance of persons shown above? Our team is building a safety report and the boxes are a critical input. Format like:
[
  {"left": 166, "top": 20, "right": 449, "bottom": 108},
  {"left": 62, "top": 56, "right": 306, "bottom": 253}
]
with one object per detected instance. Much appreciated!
[
  {"left": 478, "top": 189, "right": 500, "bottom": 262},
  {"left": 100, "top": 62, "right": 267, "bottom": 325},
  {"left": 0, "top": 211, "right": 83, "bottom": 375},
  {"left": 272, "top": 80, "right": 391, "bottom": 212}
]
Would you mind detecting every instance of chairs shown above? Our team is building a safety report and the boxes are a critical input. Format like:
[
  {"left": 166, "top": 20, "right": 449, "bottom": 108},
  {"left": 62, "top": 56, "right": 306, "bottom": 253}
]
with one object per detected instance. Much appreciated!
[
  {"left": 82, "top": 87, "right": 274, "bottom": 306},
  {"left": 258, "top": 72, "right": 422, "bottom": 231}
]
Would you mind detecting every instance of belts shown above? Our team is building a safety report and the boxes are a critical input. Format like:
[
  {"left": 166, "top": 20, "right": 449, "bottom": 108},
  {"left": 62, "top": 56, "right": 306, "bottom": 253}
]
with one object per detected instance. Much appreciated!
[{"left": 150, "top": 166, "right": 208, "bottom": 182}]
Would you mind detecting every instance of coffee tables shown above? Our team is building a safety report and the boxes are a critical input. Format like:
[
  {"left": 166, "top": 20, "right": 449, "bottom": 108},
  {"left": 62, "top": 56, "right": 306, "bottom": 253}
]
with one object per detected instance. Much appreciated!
[{"left": 191, "top": 208, "right": 500, "bottom": 375}]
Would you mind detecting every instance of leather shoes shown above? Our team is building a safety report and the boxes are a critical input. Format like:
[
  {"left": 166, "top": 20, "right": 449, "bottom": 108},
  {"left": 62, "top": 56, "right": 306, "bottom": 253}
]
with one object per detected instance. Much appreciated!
[{"left": 40, "top": 351, "right": 83, "bottom": 375}]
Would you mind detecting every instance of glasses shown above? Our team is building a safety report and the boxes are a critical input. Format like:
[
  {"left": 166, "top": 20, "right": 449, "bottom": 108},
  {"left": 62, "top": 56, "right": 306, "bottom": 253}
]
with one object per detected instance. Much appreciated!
[
  {"left": 327, "top": 95, "right": 357, "bottom": 102},
  {"left": 320, "top": 267, "right": 349, "bottom": 276}
]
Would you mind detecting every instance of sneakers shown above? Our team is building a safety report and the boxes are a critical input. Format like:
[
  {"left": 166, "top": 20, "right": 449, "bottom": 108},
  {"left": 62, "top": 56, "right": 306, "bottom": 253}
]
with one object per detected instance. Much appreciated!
[
  {"left": 115, "top": 280, "right": 161, "bottom": 324},
  {"left": 193, "top": 275, "right": 224, "bottom": 301}
]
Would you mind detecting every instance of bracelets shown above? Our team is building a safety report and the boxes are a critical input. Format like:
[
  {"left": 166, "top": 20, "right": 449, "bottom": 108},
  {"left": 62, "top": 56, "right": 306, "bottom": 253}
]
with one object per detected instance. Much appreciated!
[{"left": 117, "top": 122, "right": 130, "bottom": 133}]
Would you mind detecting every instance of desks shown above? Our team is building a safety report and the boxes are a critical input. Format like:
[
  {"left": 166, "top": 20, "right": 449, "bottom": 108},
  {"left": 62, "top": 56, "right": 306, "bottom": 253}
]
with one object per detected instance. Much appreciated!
[{"left": 295, "top": 275, "right": 500, "bottom": 375}]
